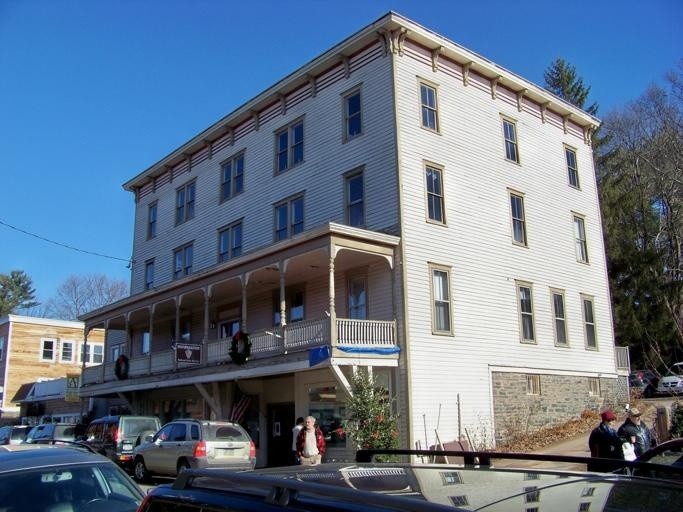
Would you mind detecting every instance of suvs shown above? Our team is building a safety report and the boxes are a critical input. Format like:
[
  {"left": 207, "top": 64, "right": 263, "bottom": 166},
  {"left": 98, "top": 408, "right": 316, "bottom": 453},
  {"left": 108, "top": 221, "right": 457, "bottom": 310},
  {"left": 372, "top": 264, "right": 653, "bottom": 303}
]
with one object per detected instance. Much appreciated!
[
  {"left": 136, "top": 450, "right": 683, "bottom": 511},
  {"left": 657, "top": 362, "right": 683, "bottom": 397},
  {"left": 0, "top": 416, "right": 257, "bottom": 512},
  {"left": 630, "top": 370, "right": 656, "bottom": 397}
]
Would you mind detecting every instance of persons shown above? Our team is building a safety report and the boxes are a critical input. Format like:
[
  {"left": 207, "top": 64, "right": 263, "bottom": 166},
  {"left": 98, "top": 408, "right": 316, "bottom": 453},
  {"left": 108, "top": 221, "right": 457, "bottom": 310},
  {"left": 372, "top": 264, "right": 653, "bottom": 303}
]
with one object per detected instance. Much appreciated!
[
  {"left": 615, "top": 407, "right": 651, "bottom": 462},
  {"left": 290, "top": 416, "right": 303, "bottom": 465},
  {"left": 98, "top": 429, "right": 117, "bottom": 462},
  {"left": 295, "top": 415, "right": 326, "bottom": 467},
  {"left": 585, "top": 409, "right": 628, "bottom": 473}
]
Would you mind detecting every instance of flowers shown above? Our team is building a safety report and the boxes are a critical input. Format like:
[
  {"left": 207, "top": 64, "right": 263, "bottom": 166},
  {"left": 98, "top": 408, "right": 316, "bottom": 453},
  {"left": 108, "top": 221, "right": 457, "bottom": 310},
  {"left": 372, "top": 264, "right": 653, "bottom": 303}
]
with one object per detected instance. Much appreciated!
[{"left": 335, "top": 365, "right": 404, "bottom": 463}]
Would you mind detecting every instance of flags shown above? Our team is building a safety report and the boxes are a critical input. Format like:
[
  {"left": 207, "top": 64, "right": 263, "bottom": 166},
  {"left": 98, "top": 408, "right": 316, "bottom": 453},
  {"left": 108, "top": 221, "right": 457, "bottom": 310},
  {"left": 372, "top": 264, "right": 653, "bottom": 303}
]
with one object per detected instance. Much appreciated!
[{"left": 228, "top": 390, "right": 251, "bottom": 424}]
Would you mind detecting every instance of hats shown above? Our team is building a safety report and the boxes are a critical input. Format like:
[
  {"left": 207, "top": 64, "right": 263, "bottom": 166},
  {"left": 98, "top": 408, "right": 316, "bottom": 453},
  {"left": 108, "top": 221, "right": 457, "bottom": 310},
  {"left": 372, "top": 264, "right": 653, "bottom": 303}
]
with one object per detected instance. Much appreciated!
[
  {"left": 629, "top": 408, "right": 643, "bottom": 417},
  {"left": 600, "top": 411, "right": 617, "bottom": 422}
]
